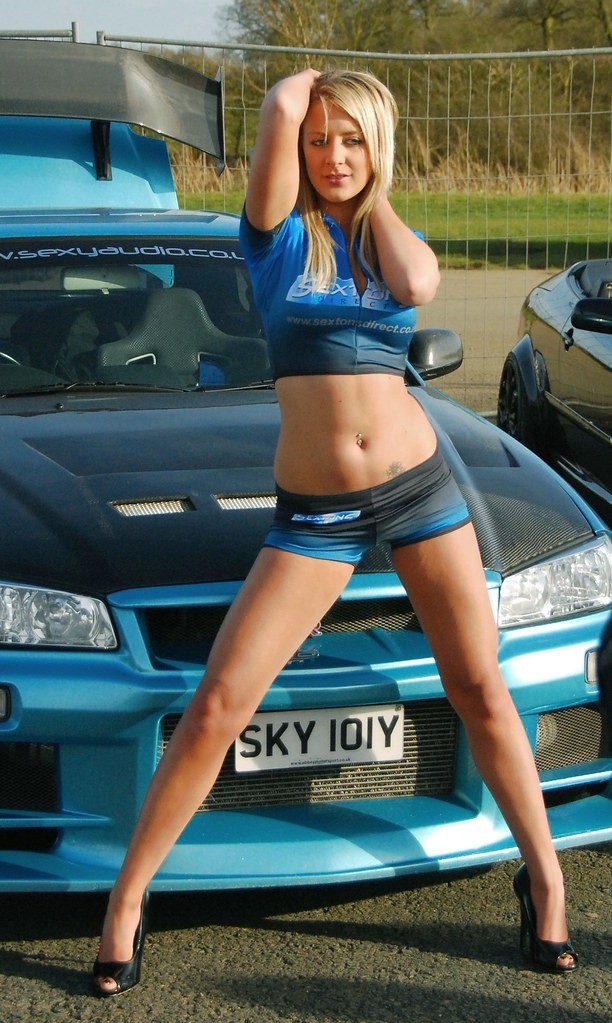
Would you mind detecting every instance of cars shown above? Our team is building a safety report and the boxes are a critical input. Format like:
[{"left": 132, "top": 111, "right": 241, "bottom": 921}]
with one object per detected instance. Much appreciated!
[
  {"left": 496, "top": 257, "right": 612, "bottom": 527},
  {"left": 0, "top": 32, "right": 612, "bottom": 894}
]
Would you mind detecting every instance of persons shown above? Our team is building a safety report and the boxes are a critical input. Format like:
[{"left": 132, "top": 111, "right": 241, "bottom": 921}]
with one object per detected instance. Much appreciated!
[{"left": 89, "top": 67, "right": 578, "bottom": 993}]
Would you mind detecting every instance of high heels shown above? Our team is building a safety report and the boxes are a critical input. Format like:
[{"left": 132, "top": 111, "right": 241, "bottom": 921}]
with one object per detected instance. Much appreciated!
[
  {"left": 514, "top": 862, "right": 580, "bottom": 973},
  {"left": 93, "top": 885, "right": 150, "bottom": 997}
]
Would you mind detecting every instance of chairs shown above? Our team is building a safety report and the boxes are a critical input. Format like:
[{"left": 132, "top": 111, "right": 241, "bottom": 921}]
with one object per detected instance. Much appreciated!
[{"left": 97, "top": 287, "right": 270, "bottom": 388}]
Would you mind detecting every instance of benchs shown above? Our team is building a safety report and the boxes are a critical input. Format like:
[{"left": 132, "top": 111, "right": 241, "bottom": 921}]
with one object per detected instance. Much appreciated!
[{"left": 0, "top": 305, "right": 130, "bottom": 372}]
